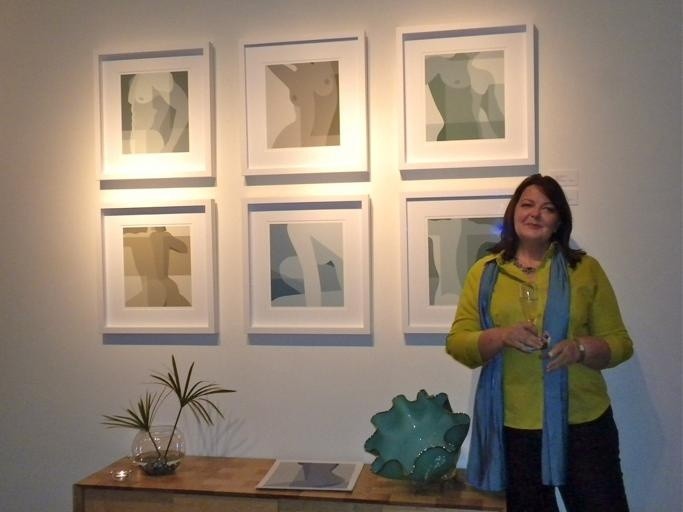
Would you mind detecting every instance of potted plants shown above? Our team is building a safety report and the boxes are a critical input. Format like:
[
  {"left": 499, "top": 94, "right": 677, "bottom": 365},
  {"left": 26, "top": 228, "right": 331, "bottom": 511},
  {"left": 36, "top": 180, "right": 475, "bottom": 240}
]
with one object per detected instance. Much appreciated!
[{"left": 98, "top": 355, "right": 236, "bottom": 476}]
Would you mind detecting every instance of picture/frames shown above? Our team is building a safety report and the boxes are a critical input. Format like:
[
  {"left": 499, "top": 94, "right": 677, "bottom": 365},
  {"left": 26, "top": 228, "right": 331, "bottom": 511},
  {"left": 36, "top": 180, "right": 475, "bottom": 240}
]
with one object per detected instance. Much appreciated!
[
  {"left": 394, "top": 21, "right": 539, "bottom": 173},
  {"left": 397, "top": 188, "right": 531, "bottom": 336},
  {"left": 88, "top": 40, "right": 218, "bottom": 182},
  {"left": 238, "top": 194, "right": 375, "bottom": 338},
  {"left": 235, "top": 29, "right": 371, "bottom": 178},
  {"left": 96, "top": 198, "right": 220, "bottom": 338}
]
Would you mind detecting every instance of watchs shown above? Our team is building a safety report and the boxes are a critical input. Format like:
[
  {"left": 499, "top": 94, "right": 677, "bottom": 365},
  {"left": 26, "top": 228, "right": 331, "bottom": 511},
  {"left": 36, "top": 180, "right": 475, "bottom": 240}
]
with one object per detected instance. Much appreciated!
[{"left": 571, "top": 338, "right": 586, "bottom": 363}]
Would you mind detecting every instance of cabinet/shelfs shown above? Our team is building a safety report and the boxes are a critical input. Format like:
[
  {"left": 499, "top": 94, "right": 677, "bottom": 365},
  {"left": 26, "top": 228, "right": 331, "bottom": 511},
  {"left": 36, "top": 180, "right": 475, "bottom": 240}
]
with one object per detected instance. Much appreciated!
[{"left": 72, "top": 451, "right": 510, "bottom": 507}]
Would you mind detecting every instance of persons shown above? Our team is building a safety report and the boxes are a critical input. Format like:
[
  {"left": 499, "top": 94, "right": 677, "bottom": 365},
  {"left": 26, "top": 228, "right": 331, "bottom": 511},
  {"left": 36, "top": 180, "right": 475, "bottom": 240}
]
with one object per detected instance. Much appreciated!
[{"left": 446, "top": 172, "right": 635, "bottom": 512}]
